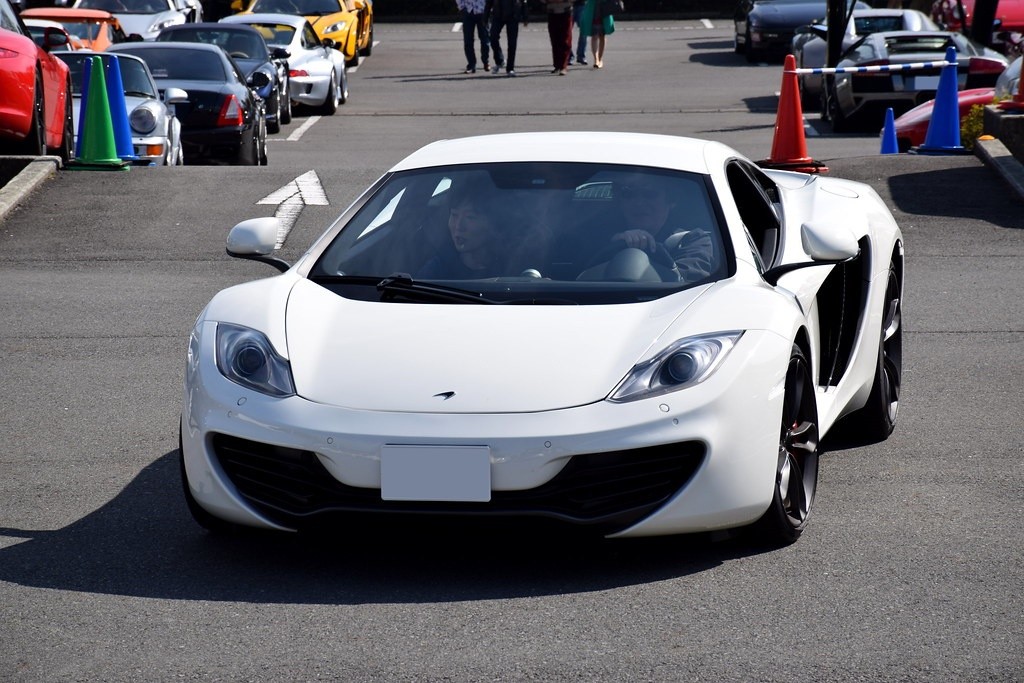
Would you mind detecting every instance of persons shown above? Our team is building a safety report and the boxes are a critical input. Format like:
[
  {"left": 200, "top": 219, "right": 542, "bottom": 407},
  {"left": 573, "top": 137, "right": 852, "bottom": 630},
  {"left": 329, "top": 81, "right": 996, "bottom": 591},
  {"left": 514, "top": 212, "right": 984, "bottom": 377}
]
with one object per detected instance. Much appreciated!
[
  {"left": 456, "top": 0, "right": 528, "bottom": 77},
  {"left": 569, "top": 173, "right": 713, "bottom": 280},
  {"left": 547, "top": 0, "right": 615, "bottom": 74},
  {"left": 413, "top": 176, "right": 523, "bottom": 280}
]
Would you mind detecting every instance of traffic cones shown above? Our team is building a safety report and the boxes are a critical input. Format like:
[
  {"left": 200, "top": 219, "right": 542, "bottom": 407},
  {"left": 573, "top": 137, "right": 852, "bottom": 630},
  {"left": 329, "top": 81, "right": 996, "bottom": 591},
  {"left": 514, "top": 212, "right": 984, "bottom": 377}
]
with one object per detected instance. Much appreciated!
[
  {"left": 907, "top": 45, "right": 967, "bottom": 157},
  {"left": 765, "top": 53, "right": 830, "bottom": 174},
  {"left": 880, "top": 107, "right": 899, "bottom": 155}
]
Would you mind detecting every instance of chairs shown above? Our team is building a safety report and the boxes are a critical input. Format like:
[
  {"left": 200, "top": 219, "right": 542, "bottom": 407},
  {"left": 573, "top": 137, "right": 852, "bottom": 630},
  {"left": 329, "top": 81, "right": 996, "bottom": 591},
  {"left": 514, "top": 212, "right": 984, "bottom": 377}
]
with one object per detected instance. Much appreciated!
[{"left": 275, "top": 31, "right": 294, "bottom": 44}]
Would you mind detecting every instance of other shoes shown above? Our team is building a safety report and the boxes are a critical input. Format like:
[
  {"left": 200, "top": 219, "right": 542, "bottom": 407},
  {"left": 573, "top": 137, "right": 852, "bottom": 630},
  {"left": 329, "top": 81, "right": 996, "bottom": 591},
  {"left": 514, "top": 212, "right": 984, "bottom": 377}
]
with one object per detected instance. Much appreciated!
[
  {"left": 465, "top": 69, "right": 473, "bottom": 73},
  {"left": 594, "top": 62, "right": 599, "bottom": 67},
  {"left": 484, "top": 63, "right": 490, "bottom": 70},
  {"left": 577, "top": 57, "right": 587, "bottom": 65},
  {"left": 507, "top": 70, "right": 516, "bottom": 77},
  {"left": 568, "top": 55, "right": 573, "bottom": 64},
  {"left": 560, "top": 68, "right": 567, "bottom": 75},
  {"left": 551, "top": 69, "right": 559, "bottom": 73},
  {"left": 492, "top": 62, "right": 504, "bottom": 73},
  {"left": 598, "top": 62, "right": 602, "bottom": 68}
]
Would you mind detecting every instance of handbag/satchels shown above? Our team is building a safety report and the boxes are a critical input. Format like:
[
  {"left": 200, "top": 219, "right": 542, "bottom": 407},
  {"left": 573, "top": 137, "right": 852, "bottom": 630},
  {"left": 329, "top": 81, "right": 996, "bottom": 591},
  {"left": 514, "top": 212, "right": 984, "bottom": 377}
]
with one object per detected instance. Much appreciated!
[{"left": 606, "top": 0, "right": 624, "bottom": 14}]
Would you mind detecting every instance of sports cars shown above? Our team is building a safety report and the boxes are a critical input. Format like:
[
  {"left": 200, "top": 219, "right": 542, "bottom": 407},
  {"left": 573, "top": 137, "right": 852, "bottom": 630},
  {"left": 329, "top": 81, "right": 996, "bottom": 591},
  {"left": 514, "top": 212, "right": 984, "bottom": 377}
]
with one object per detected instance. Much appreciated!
[
  {"left": 212, "top": 11, "right": 349, "bottom": 115},
  {"left": 174, "top": 126, "right": 911, "bottom": 557}
]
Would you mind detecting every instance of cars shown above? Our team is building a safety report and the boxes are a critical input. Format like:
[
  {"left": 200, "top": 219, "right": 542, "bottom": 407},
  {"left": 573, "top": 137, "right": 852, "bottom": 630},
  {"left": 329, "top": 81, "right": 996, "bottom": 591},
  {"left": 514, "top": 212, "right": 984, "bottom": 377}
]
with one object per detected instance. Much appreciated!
[
  {"left": 101, "top": 40, "right": 271, "bottom": 167},
  {"left": 733, "top": 0, "right": 1024, "bottom": 156},
  {"left": 151, "top": 20, "right": 293, "bottom": 135},
  {"left": 1, "top": 0, "right": 375, "bottom": 175}
]
